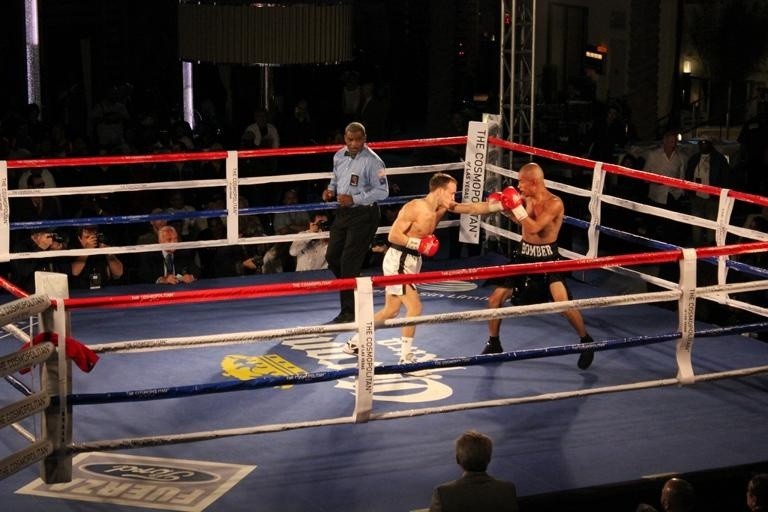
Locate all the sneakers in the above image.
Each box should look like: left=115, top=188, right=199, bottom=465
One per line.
left=481, top=341, right=502, bottom=355
left=343, top=339, right=358, bottom=355
left=577, top=350, right=594, bottom=370
left=333, top=312, right=354, bottom=322
left=398, top=358, right=425, bottom=377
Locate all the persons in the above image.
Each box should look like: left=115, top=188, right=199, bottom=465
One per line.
left=428, top=428, right=519, bottom=511
left=744, top=471, right=768, bottom=511
left=0, top=68, right=767, bottom=290
left=476, top=160, right=599, bottom=370
left=660, top=473, right=695, bottom=511
left=321, top=122, right=390, bottom=327
left=343, top=171, right=509, bottom=371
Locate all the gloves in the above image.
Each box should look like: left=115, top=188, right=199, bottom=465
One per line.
left=405, top=235, right=439, bottom=256
left=487, top=186, right=528, bottom=222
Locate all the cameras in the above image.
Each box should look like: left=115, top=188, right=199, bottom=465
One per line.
left=51, top=233, right=65, bottom=242
left=94, top=231, right=104, bottom=244
left=313, top=220, right=330, bottom=230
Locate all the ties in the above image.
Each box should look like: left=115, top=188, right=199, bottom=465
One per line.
left=167, top=253, right=173, bottom=274
